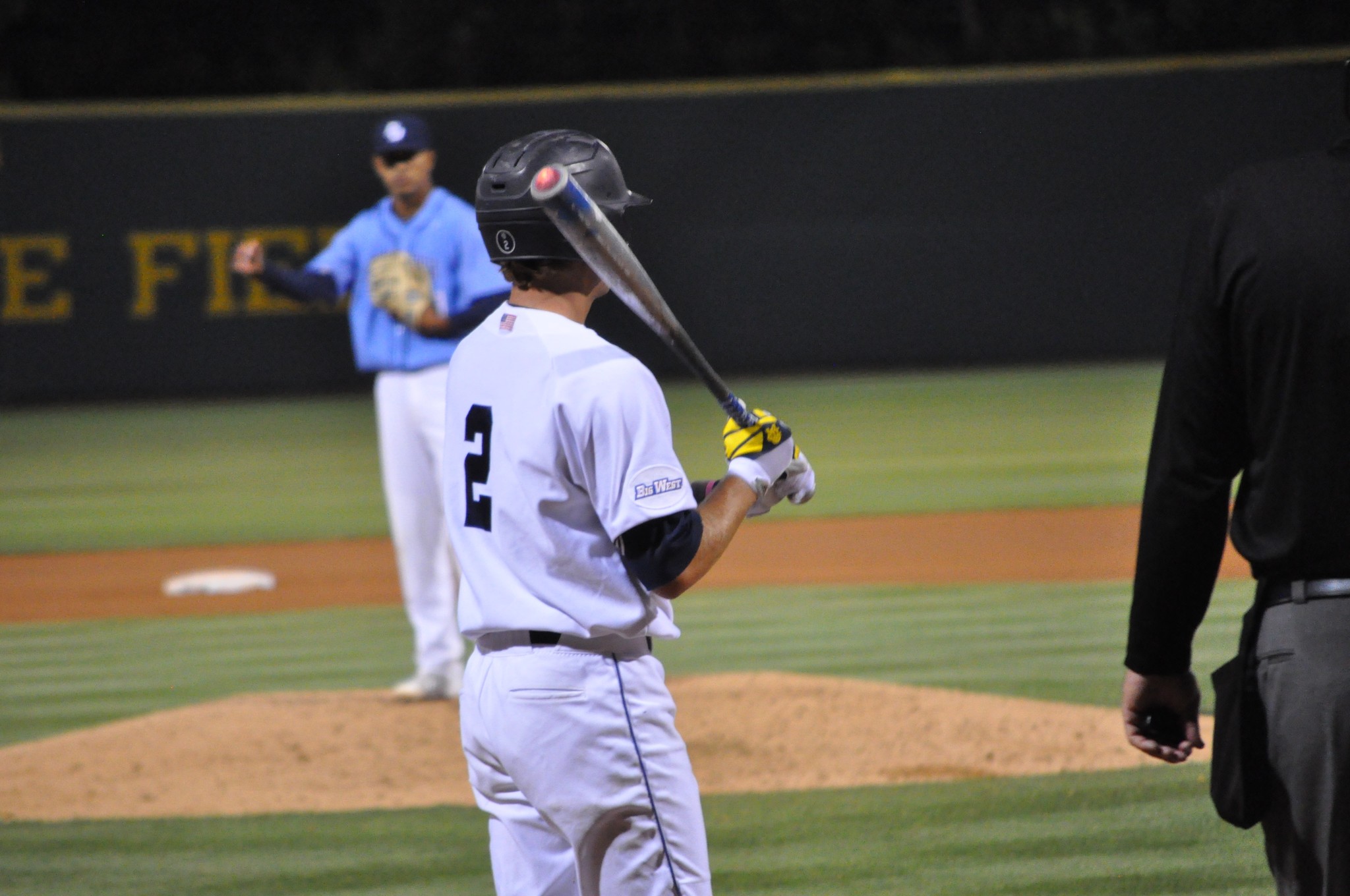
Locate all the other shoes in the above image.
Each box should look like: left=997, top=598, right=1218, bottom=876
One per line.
left=394, top=678, right=443, bottom=698
left=443, top=671, right=461, bottom=697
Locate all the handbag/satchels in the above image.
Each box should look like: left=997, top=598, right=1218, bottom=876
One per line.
left=1211, top=605, right=1273, bottom=830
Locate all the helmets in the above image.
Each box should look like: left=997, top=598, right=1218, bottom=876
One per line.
left=476, top=129, right=654, bottom=263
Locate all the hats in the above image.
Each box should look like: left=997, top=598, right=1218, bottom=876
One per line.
left=371, top=115, right=432, bottom=155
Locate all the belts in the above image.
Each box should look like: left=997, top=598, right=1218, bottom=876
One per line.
left=527, top=630, right=654, bottom=651
left=1253, top=576, right=1349, bottom=606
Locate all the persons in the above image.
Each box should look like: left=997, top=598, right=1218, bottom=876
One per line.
left=1121, top=147, right=1350, bottom=896
left=230, top=112, right=514, bottom=701
left=439, top=127, right=817, bottom=896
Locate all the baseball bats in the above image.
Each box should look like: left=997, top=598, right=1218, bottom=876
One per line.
left=531, top=162, right=758, bottom=431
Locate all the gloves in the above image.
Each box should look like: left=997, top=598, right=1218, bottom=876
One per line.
left=744, top=446, right=814, bottom=517
left=723, top=397, right=796, bottom=495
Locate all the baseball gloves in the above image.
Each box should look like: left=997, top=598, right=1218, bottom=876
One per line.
left=370, top=250, right=437, bottom=333
